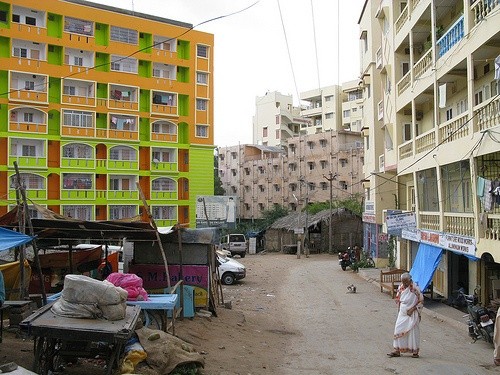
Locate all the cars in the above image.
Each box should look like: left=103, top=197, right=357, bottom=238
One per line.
left=216, top=252, right=246, bottom=285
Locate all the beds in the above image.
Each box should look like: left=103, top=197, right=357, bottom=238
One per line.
left=379, top=270, right=434, bottom=300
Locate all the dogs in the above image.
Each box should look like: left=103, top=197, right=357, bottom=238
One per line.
left=348, top=283, right=356, bottom=293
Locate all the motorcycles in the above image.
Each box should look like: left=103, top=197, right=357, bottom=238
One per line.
left=460, top=289, right=495, bottom=345
left=338, top=249, right=355, bottom=271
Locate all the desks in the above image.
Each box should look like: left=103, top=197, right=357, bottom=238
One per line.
left=3, top=301, right=32, bottom=328
left=18, top=302, right=141, bottom=375
left=46, top=291, right=178, bottom=337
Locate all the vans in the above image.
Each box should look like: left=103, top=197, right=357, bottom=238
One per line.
left=218, top=233, right=247, bottom=258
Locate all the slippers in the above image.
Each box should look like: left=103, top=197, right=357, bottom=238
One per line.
left=412, top=354, right=419, bottom=358
left=386, top=352, right=401, bottom=357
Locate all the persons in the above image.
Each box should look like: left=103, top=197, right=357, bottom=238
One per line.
left=387, top=272, right=424, bottom=358
left=493, top=307, right=500, bottom=366
